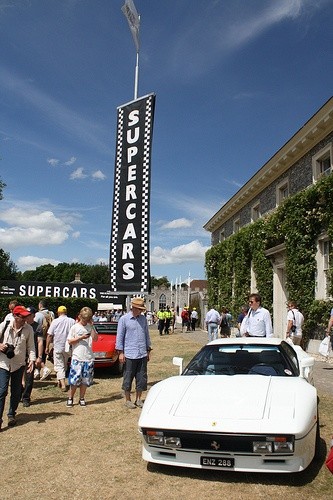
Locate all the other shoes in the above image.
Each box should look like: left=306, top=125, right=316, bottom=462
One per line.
left=125, top=399, right=136, bottom=409
left=134, top=399, right=144, bottom=408
left=66, top=397, right=74, bottom=408
left=57, top=383, right=67, bottom=392
left=7, top=414, right=17, bottom=427
left=23, top=398, right=31, bottom=407
left=78, top=398, right=86, bottom=407
left=40, top=367, right=52, bottom=382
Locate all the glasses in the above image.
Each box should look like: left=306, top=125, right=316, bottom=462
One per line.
left=249, top=300, right=255, bottom=304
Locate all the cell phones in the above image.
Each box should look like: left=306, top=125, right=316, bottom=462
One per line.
left=86, top=333, right=90, bottom=335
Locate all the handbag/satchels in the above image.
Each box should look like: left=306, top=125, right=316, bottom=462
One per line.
left=318, top=336, right=330, bottom=357
left=290, top=324, right=296, bottom=332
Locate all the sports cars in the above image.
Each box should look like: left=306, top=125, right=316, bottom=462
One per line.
left=140, top=337, right=319, bottom=474
left=67, top=322, right=125, bottom=374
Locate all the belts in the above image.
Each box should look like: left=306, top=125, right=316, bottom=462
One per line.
left=210, top=322, right=217, bottom=323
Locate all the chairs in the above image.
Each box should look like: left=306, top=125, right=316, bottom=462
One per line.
left=259, top=350, right=287, bottom=375
left=205, top=352, right=235, bottom=375
left=231, top=350, right=254, bottom=374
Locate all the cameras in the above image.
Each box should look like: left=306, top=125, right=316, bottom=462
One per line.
left=291, top=325, right=296, bottom=332
left=2, top=343, right=15, bottom=359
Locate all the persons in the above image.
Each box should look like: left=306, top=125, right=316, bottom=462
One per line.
left=285, top=300, right=304, bottom=345
left=326, top=308, right=333, bottom=352
left=115, top=297, right=151, bottom=408
left=67, top=306, right=99, bottom=408
left=206, top=304, right=247, bottom=342
left=0, top=300, right=77, bottom=431
left=240, top=294, right=272, bottom=338
left=147, top=307, right=198, bottom=336
left=94, top=308, right=128, bottom=323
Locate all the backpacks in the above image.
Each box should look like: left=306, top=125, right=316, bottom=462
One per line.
left=39, top=310, right=54, bottom=336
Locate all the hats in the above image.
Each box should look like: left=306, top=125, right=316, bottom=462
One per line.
left=56, top=306, right=67, bottom=313
left=129, top=298, right=147, bottom=310
left=193, top=308, right=196, bottom=311
left=13, top=306, right=31, bottom=317
